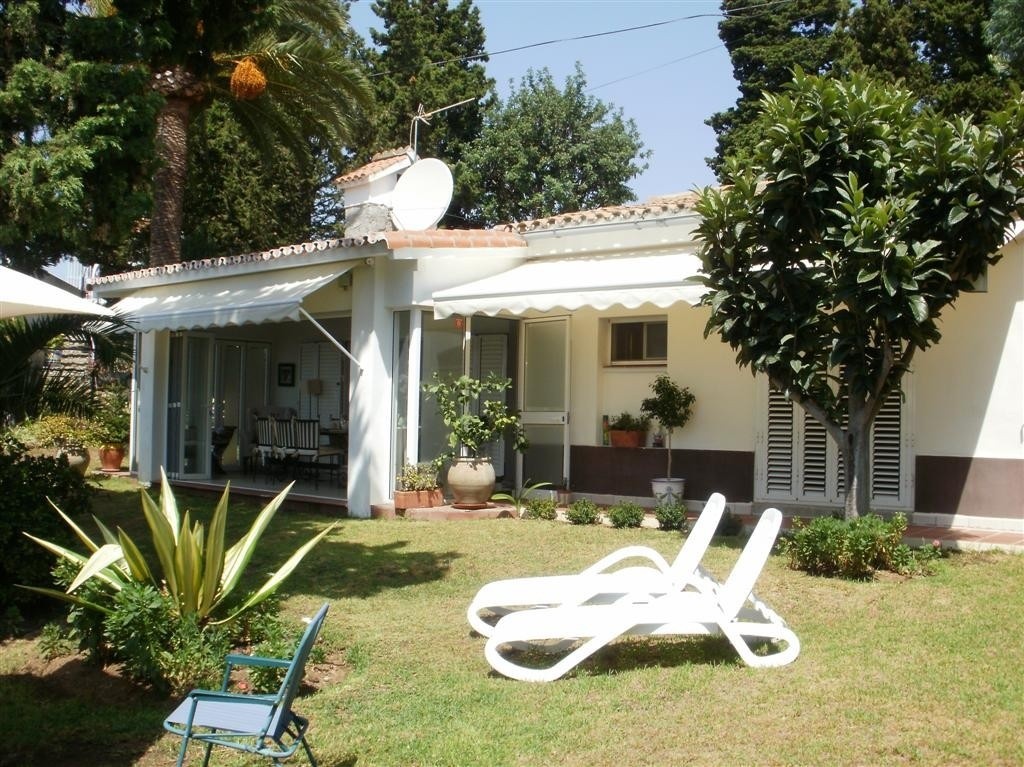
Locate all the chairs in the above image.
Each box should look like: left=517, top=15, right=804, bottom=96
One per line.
left=465, top=491, right=804, bottom=688
left=254, top=409, right=342, bottom=490
left=162, top=599, right=331, bottom=767
left=181, top=419, right=240, bottom=481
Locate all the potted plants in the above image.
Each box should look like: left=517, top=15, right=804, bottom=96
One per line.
left=420, top=366, right=532, bottom=510
left=639, top=372, right=699, bottom=511
left=93, top=391, right=131, bottom=472
left=394, top=455, right=446, bottom=512
left=606, top=411, right=651, bottom=447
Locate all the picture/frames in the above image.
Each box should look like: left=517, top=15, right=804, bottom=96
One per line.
left=277, top=364, right=298, bottom=388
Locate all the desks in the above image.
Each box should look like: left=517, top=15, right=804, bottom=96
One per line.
left=317, top=426, right=349, bottom=485
